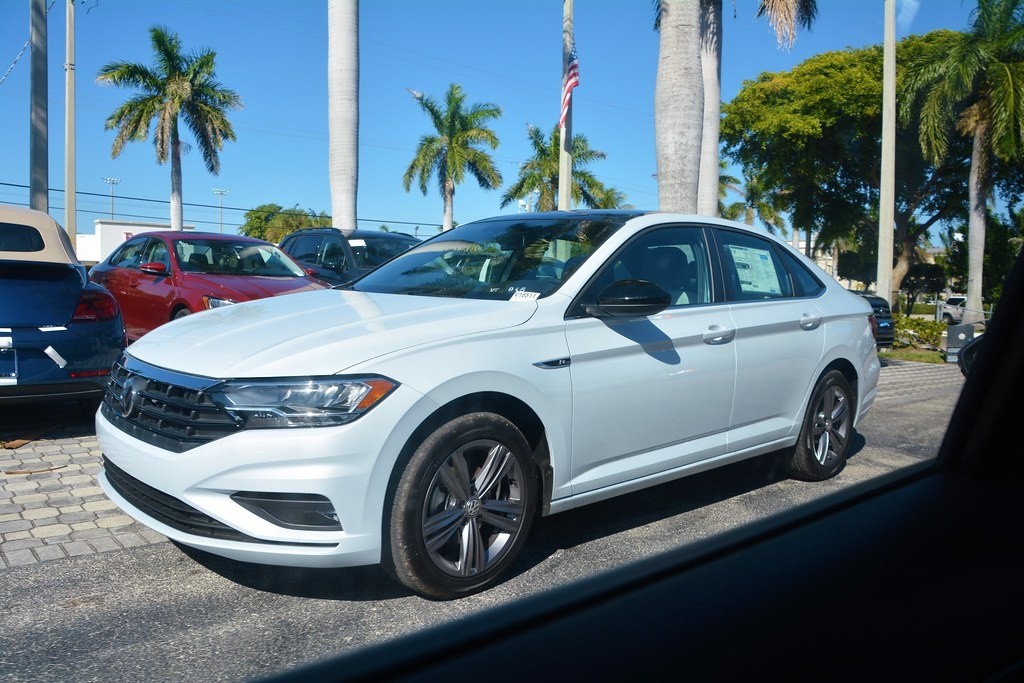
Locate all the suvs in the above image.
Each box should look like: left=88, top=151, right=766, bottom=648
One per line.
left=277, top=227, right=460, bottom=291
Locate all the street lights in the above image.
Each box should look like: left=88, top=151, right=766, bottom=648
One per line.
left=210, top=188, right=230, bottom=234
left=102, top=177, right=122, bottom=220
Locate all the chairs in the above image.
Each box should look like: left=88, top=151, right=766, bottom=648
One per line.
left=134, top=251, right=153, bottom=264
left=189, top=253, right=210, bottom=273
left=513, top=248, right=695, bottom=309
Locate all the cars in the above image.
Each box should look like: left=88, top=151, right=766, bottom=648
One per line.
left=846, top=289, right=896, bottom=349
left=85, top=230, right=332, bottom=345
left=927, top=296, right=968, bottom=325
left=92, top=210, right=883, bottom=602
left=0, top=202, right=129, bottom=405
